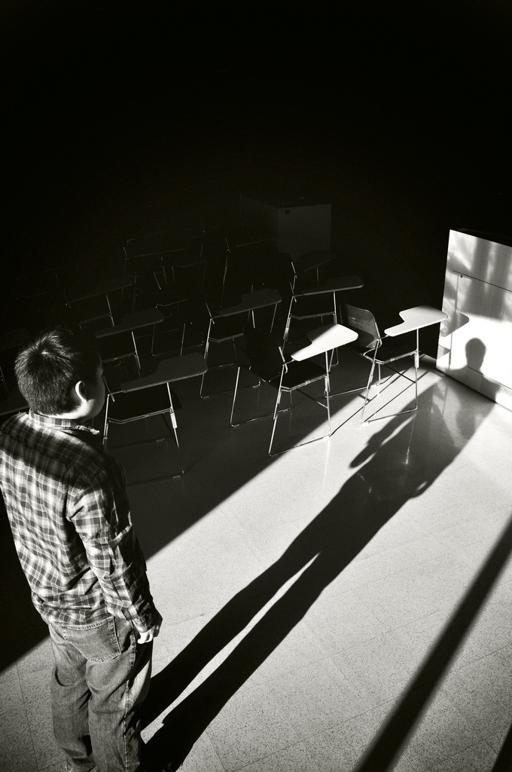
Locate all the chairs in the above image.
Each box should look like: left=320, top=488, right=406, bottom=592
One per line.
left=1, top=258, right=448, bottom=486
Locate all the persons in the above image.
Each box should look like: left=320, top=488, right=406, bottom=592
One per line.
left=1, top=322, right=166, bottom=772
